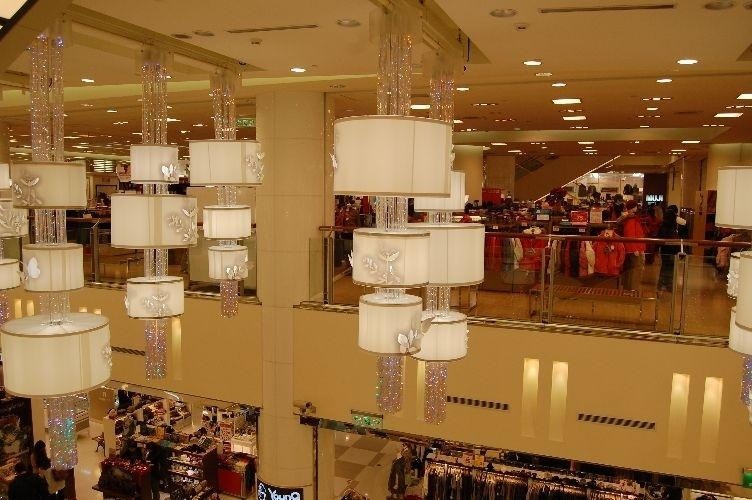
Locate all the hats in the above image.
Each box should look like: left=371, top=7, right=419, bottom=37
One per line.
left=626, top=200, right=637, bottom=210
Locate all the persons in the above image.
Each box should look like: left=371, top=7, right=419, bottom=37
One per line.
left=36, top=456, right=70, bottom=499
left=31, top=438, right=47, bottom=474
left=146, top=439, right=163, bottom=497
left=618, top=201, right=644, bottom=291
left=6, top=461, right=50, bottom=500
left=116, top=389, right=130, bottom=412
left=116, top=436, right=144, bottom=460
left=639, top=202, right=690, bottom=292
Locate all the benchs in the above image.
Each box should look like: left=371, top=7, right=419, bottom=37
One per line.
left=529, top=284, right=660, bottom=332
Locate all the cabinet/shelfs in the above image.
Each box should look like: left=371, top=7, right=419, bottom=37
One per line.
left=0, top=399, right=36, bottom=477
left=91, top=389, right=258, bottom=500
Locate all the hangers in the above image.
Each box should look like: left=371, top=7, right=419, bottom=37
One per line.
left=523, top=216, right=540, bottom=234
left=421, top=456, right=645, bottom=500
left=602, top=225, right=616, bottom=237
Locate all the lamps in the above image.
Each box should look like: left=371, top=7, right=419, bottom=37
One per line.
left=1, top=29, right=114, bottom=469
left=111, top=45, right=198, bottom=379
left=714, top=166, right=751, bottom=425
left=331, top=1, right=454, bottom=411
left=186, top=68, right=265, bottom=318
left=405, top=44, right=486, bottom=427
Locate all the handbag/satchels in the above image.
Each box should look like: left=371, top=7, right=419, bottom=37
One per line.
left=51, top=469, right=68, bottom=481
